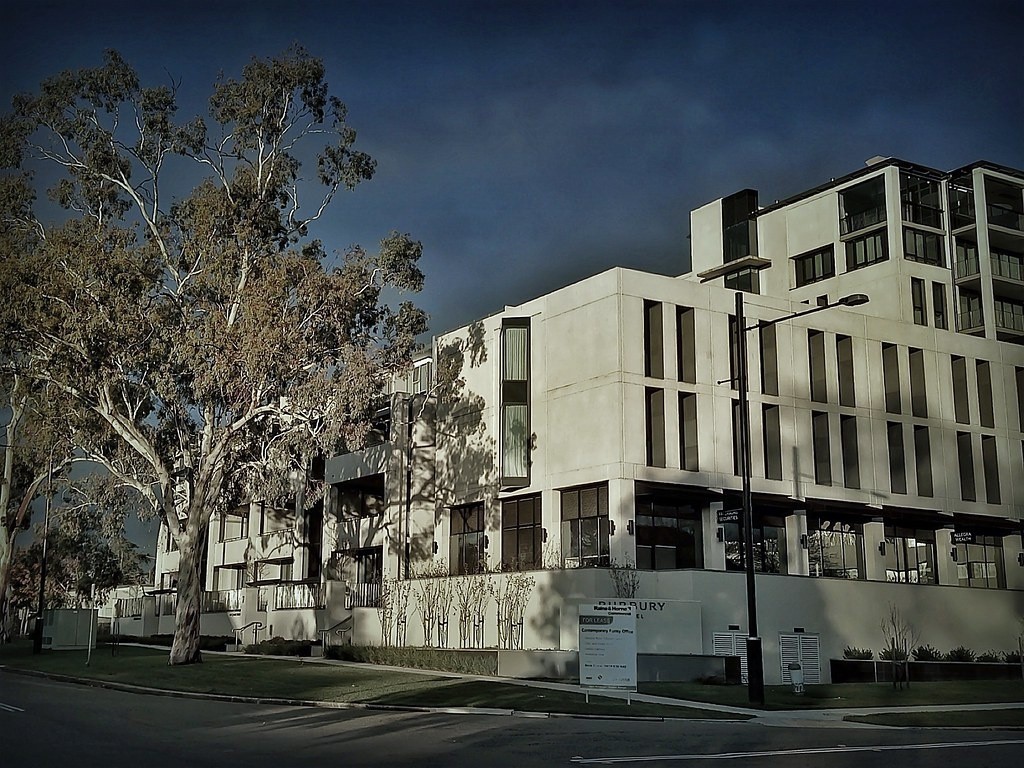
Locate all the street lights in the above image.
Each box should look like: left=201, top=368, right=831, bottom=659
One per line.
left=716, top=292, right=871, bottom=708
